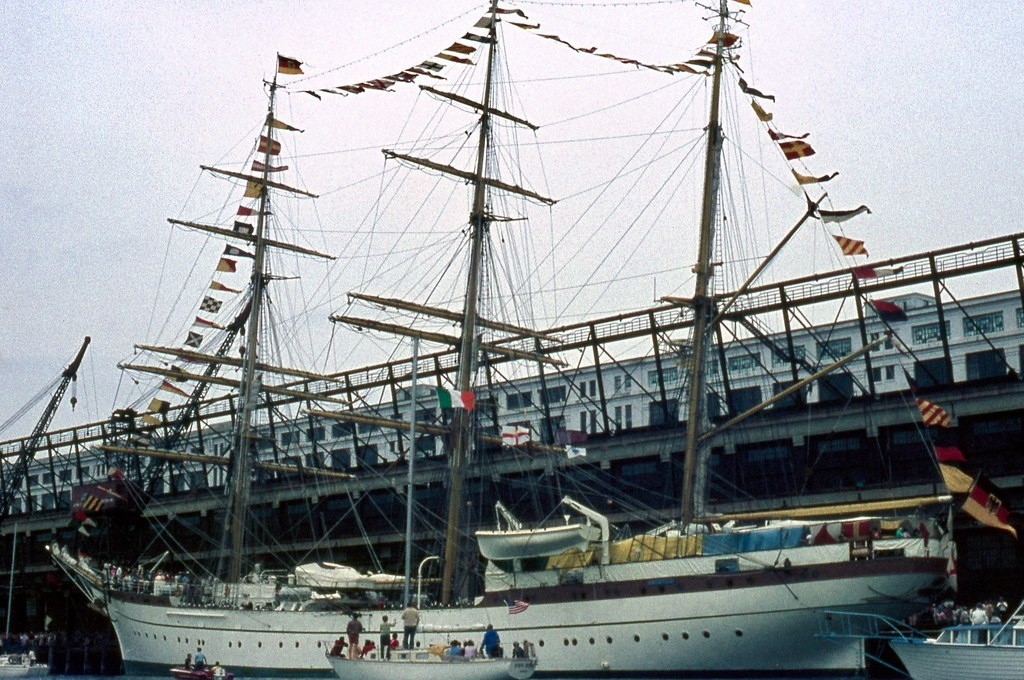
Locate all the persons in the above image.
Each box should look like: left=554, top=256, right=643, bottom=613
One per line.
left=800, top=527, right=912, bottom=545
left=928, top=596, right=1008, bottom=626
left=102, top=563, right=217, bottom=605
left=185, top=647, right=223, bottom=673
left=2, top=630, right=106, bottom=647
left=328, top=602, right=524, bottom=659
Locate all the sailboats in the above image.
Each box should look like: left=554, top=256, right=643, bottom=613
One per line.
left=49, top=0, right=958, bottom=680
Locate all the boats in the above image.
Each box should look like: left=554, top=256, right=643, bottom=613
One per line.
left=812, top=608, right=1024, bottom=680
left=474, top=525, right=601, bottom=560
left=0, top=650, right=49, bottom=677
left=169, top=666, right=234, bottom=680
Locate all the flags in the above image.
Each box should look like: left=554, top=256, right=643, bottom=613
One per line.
left=144, top=258, right=237, bottom=414
left=393, top=71, right=419, bottom=81
left=871, top=299, right=908, bottom=322
left=445, top=42, right=476, bottom=55
left=337, top=85, right=365, bottom=95
left=539, top=27, right=742, bottom=77
left=504, top=599, right=529, bottom=615
left=233, top=220, right=254, bottom=235
left=916, top=396, right=1018, bottom=535
left=739, top=79, right=816, bottom=161
left=83, top=466, right=123, bottom=511
left=437, top=385, right=586, bottom=459
left=240, top=54, right=305, bottom=198
left=416, top=60, right=446, bottom=71
left=140, top=414, right=161, bottom=426
left=474, top=16, right=502, bottom=28
left=367, top=78, right=395, bottom=89
left=835, top=237, right=871, bottom=258
